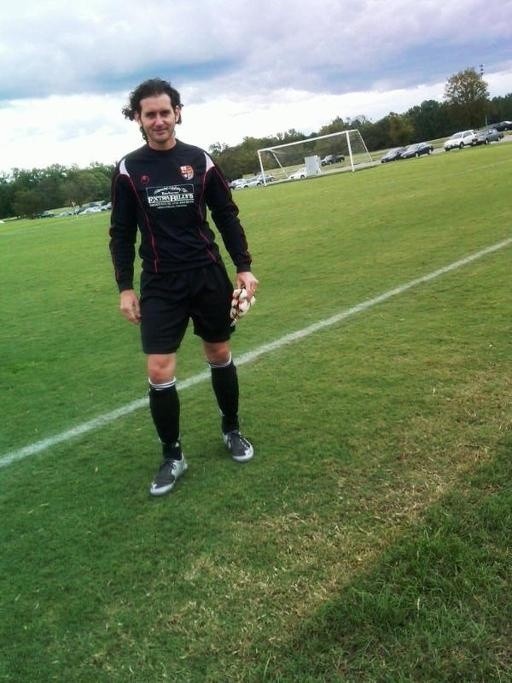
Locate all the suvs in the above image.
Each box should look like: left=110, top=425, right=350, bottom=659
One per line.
left=444, top=129, right=478, bottom=151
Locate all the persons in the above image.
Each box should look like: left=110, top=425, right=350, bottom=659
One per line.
left=107, top=74, right=262, bottom=498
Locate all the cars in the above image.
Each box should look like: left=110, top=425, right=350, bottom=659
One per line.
left=471, top=128, right=504, bottom=146
left=229, top=166, right=321, bottom=192
left=321, top=154, right=344, bottom=166
left=494, top=120, right=512, bottom=131
left=381, top=147, right=407, bottom=163
left=401, top=142, right=434, bottom=159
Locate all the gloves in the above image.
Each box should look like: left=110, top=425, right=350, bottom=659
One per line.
left=230, top=288, right=255, bottom=327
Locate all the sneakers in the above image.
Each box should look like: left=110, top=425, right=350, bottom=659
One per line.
left=222, top=429, right=254, bottom=462
left=149, top=450, right=188, bottom=496
